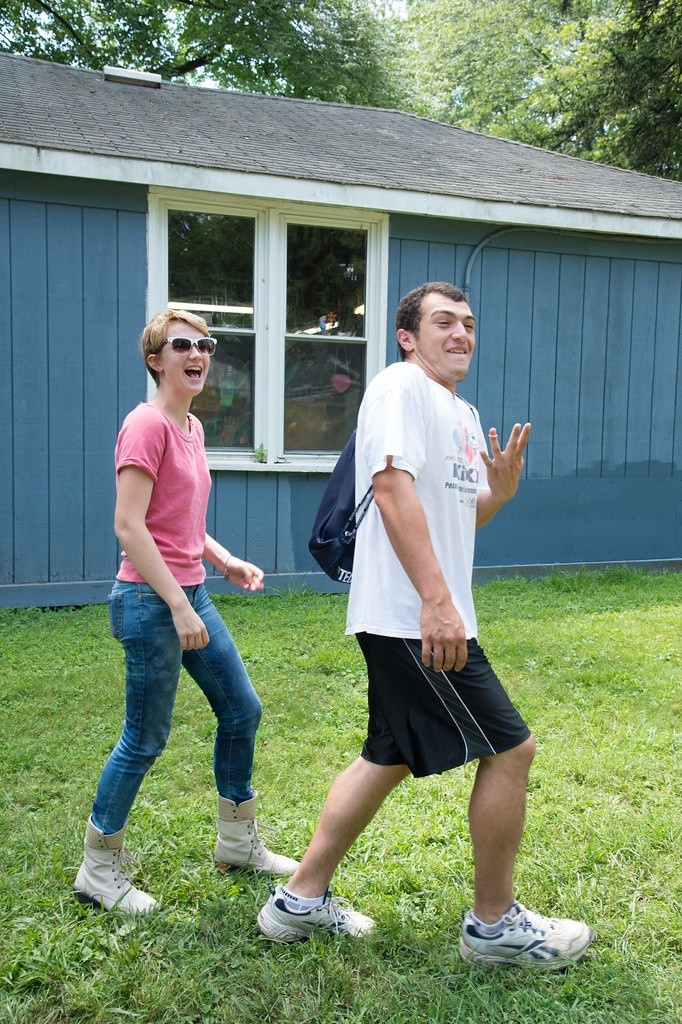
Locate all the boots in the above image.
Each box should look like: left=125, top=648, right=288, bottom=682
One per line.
left=72, top=812, right=161, bottom=915
left=215, top=791, right=300, bottom=876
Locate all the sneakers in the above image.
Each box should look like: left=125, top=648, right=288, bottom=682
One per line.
left=459, top=901, right=590, bottom=972
left=258, top=882, right=377, bottom=943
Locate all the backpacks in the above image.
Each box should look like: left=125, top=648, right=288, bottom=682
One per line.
left=308, top=427, right=376, bottom=583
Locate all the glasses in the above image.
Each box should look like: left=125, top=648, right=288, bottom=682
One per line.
left=155, top=337, right=219, bottom=357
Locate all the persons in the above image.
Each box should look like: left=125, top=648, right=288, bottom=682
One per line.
left=72, top=309, right=300, bottom=913
left=257, top=281, right=593, bottom=970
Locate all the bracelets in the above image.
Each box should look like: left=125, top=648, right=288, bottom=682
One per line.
left=223, top=555, right=232, bottom=581
